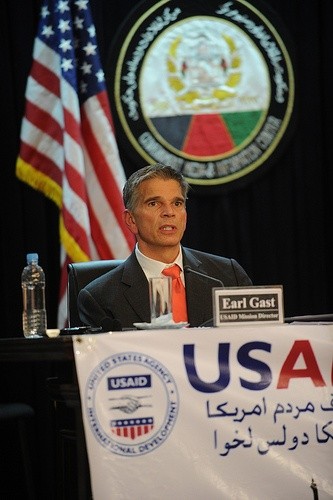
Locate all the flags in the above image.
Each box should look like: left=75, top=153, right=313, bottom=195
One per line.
left=16, top=0, right=137, bottom=329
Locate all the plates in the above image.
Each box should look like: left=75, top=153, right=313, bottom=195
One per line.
left=133, top=322, right=190, bottom=330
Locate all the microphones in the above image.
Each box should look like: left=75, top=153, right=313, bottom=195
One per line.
left=183, top=265, right=225, bottom=287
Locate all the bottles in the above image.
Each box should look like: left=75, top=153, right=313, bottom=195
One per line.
left=21, top=253, right=47, bottom=339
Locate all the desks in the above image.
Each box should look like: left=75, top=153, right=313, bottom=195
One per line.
left=0, top=324, right=333, bottom=500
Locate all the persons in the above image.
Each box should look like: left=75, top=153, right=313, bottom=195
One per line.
left=76, top=163, right=254, bottom=332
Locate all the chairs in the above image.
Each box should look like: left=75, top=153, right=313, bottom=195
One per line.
left=45, top=260, right=119, bottom=500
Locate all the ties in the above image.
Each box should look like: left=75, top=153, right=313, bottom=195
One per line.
left=162, top=265, right=188, bottom=325
